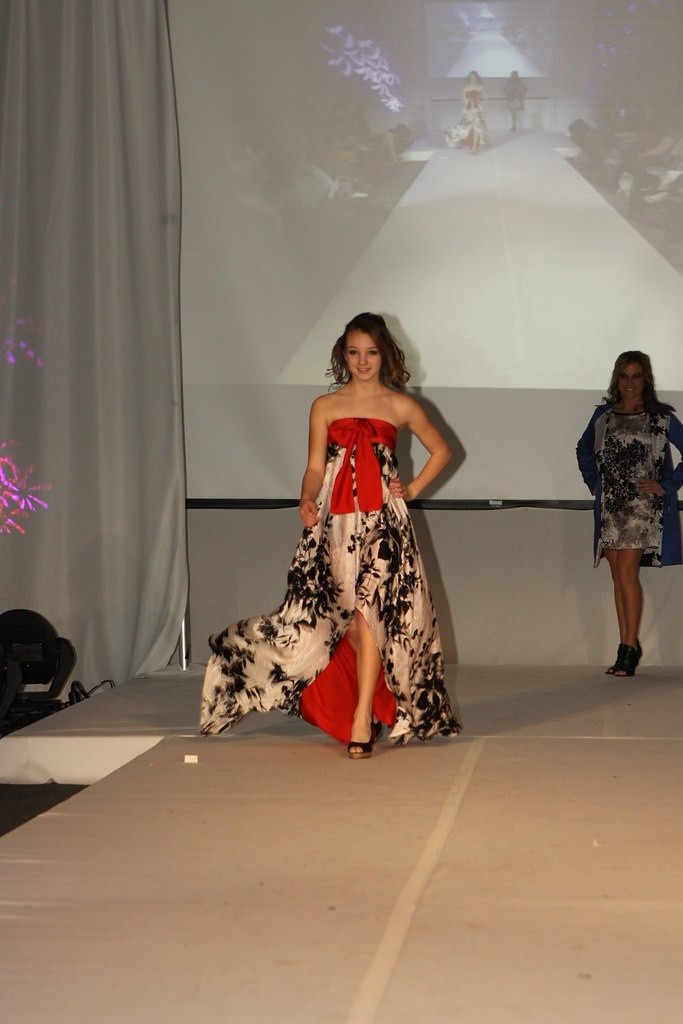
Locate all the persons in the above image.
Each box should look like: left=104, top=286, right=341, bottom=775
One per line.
left=444, top=72, right=489, bottom=155
left=201, top=117, right=413, bottom=352
left=575, top=351, right=683, bottom=677
left=568, top=104, right=683, bottom=267
left=503, top=70, right=526, bottom=135
left=197, top=312, right=462, bottom=759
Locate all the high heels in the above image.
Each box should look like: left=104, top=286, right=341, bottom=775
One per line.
left=605, top=643, right=628, bottom=674
left=347, top=714, right=383, bottom=759
left=614, top=639, right=642, bottom=676
